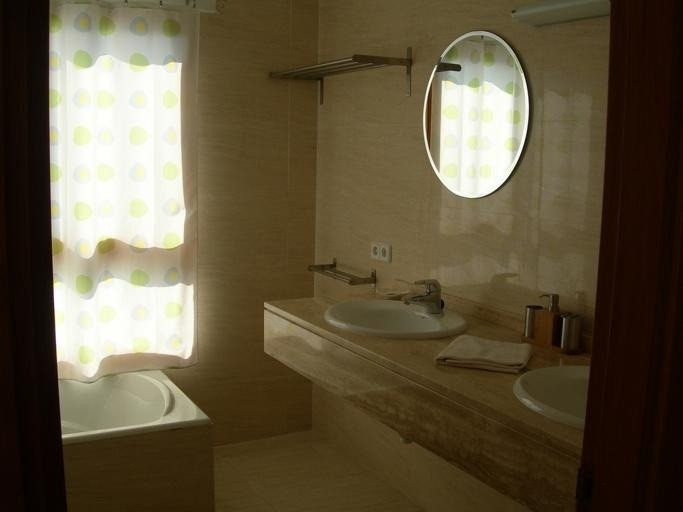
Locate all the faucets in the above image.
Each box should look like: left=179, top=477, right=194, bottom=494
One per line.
left=404, top=280, right=446, bottom=314
left=489, top=273, right=521, bottom=294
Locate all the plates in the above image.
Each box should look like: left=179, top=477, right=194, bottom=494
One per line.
left=375, top=286, right=411, bottom=298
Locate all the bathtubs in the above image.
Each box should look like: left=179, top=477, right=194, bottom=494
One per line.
left=57, top=370, right=229, bottom=447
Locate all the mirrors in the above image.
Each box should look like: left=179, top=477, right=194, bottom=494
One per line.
left=422, top=30, right=530, bottom=198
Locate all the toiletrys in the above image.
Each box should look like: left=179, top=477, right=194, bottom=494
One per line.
left=571, top=290, right=590, bottom=319
left=534, top=294, right=566, bottom=349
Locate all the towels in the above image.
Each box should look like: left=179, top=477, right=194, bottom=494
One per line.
left=434, top=334, right=536, bottom=373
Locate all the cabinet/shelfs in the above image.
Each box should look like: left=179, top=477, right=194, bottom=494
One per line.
left=269, top=47, right=412, bottom=298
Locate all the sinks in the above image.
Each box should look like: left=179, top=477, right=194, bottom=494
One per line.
left=325, top=298, right=468, bottom=340
left=513, top=364, right=590, bottom=430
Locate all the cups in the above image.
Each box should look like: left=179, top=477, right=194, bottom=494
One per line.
left=561, top=315, right=582, bottom=356
left=524, top=305, right=543, bottom=339
left=534, top=309, right=553, bottom=348
